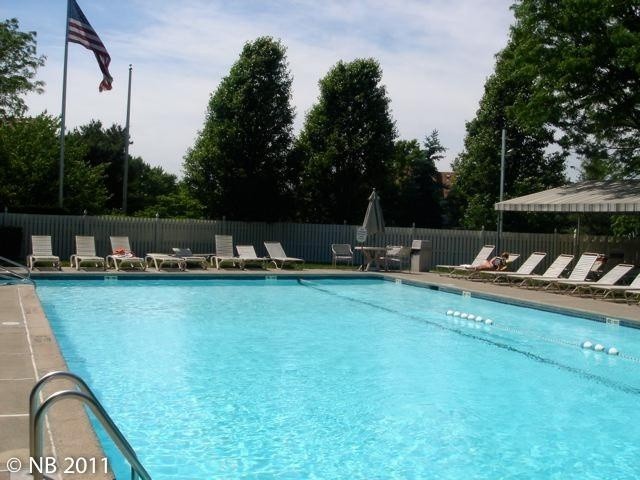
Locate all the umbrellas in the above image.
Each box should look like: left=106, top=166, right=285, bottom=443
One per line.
left=363, top=187, right=388, bottom=258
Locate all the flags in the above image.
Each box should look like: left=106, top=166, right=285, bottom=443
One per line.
left=66, top=0, right=115, bottom=92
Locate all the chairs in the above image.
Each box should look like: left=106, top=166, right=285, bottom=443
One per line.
left=208, top=232, right=241, bottom=272
left=171, top=246, right=207, bottom=271
left=264, top=241, right=306, bottom=271
left=324, top=234, right=640, bottom=309
left=102, top=234, right=146, bottom=273
left=26, top=234, right=62, bottom=272
left=144, top=252, right=184, bottom=273
left=69, top=235, right=107, bottom=273
left=236, top=244, right=269, bottom=271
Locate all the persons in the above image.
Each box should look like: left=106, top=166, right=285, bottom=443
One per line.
left=465, top=251, right=511, bottom=272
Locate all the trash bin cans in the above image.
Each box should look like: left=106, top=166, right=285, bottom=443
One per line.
left=410, top=240, right=433, bottom=272
left=0, top=227, right=23, bottom=267
